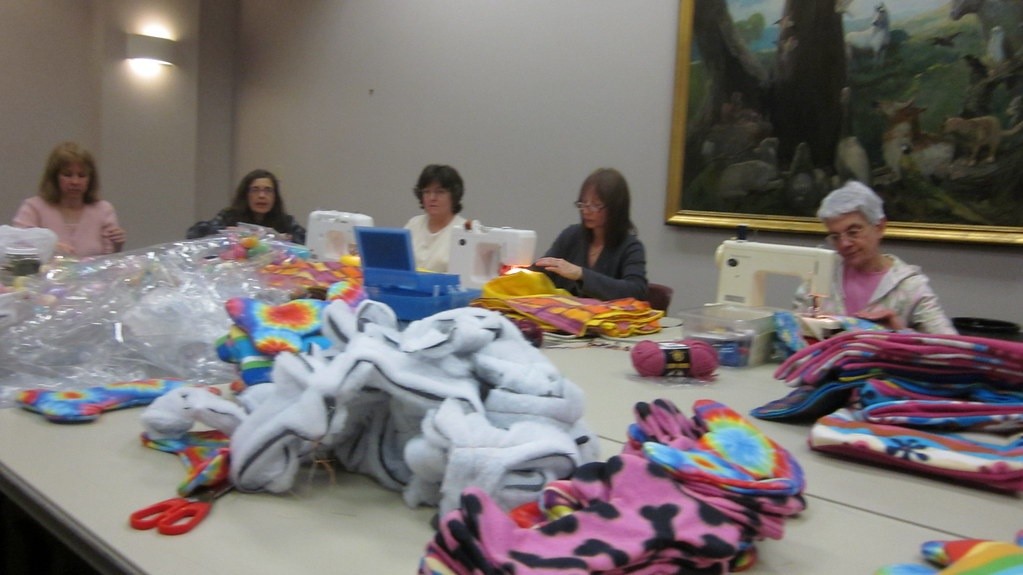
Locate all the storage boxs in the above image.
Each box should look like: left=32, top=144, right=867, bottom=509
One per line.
left=678, top=303, right=773, bottom=369
left=354, top=225, right=486, bottom=322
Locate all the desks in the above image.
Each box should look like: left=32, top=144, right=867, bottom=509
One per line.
left=0, top=318, right=1023, bottom=575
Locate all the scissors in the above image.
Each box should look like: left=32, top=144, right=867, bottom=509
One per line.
left=127, top=479, right=236, bottom=537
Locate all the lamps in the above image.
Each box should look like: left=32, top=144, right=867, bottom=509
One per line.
left=125, top=22, right=184, bottom=82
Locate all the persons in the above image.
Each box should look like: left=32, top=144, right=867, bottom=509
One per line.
left=11, top=140, right=128, bottom=256
left=185, top=169, right=306, bottom=245
left=401, top=164, right=473, bottom=272
left=789, top=180, right=959, bottom=335
left=465, top=167, right=649, bottom=301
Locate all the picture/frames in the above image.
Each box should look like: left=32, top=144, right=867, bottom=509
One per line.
left=664, top=0, right=1023, bottom=247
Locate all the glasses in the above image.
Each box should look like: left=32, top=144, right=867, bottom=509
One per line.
left=574, top=200, right=606, bottom=212
left=825, top=224, right=872, bottom=245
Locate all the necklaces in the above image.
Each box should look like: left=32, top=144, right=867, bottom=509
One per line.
left=589, top=250, right=597, bottom=255
